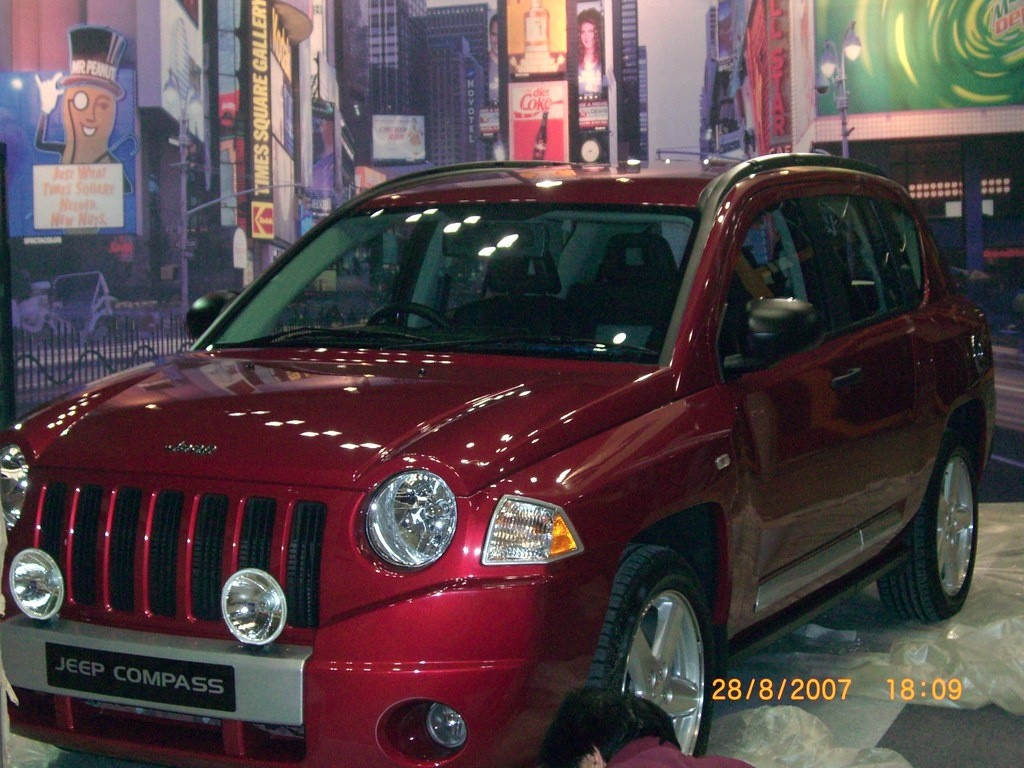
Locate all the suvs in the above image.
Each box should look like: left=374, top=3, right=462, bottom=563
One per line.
left=0, top=153, right=999, bottom=768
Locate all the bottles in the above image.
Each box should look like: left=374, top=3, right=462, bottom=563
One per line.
left=523, top=1, right=549, bottom=60
left=533, top=111, right=548, bottom=162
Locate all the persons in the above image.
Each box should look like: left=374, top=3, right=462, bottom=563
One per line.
left=537, top=685, right=755, bottom=768
left=574, top=9, right=603, bottom=96
left=487, top=15, right=499, bottom=101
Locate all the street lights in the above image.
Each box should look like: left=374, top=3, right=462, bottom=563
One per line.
left=163, top=68, right=204, bottom=328
left=813, top=20, right=862, bottom=158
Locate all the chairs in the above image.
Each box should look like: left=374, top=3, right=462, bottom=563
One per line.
left=576, top=230, right=691, bottom=350
left=451, top=224, right=590, bottom=340
